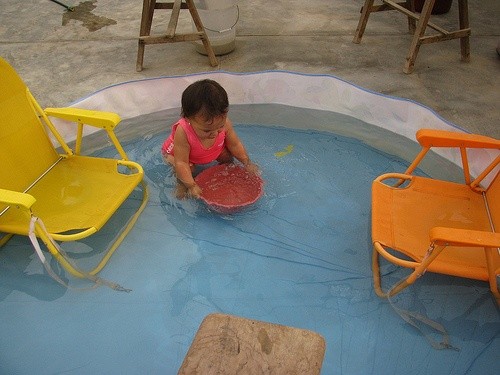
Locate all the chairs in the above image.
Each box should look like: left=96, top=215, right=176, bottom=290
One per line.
left=173, top=314, right=326, bottom=374
left=0, top=59, right=149, bottom=278
left=366, top=129, right=499, bottom=313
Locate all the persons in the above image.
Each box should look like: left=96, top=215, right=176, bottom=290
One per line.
left=161, top=79, right=262, bottom=199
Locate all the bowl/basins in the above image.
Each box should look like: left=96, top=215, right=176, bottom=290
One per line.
left=193, top=163, right=264, bottom=214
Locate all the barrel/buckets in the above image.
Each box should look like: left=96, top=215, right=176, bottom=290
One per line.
left=190, top=0, right=237, bottom=56
